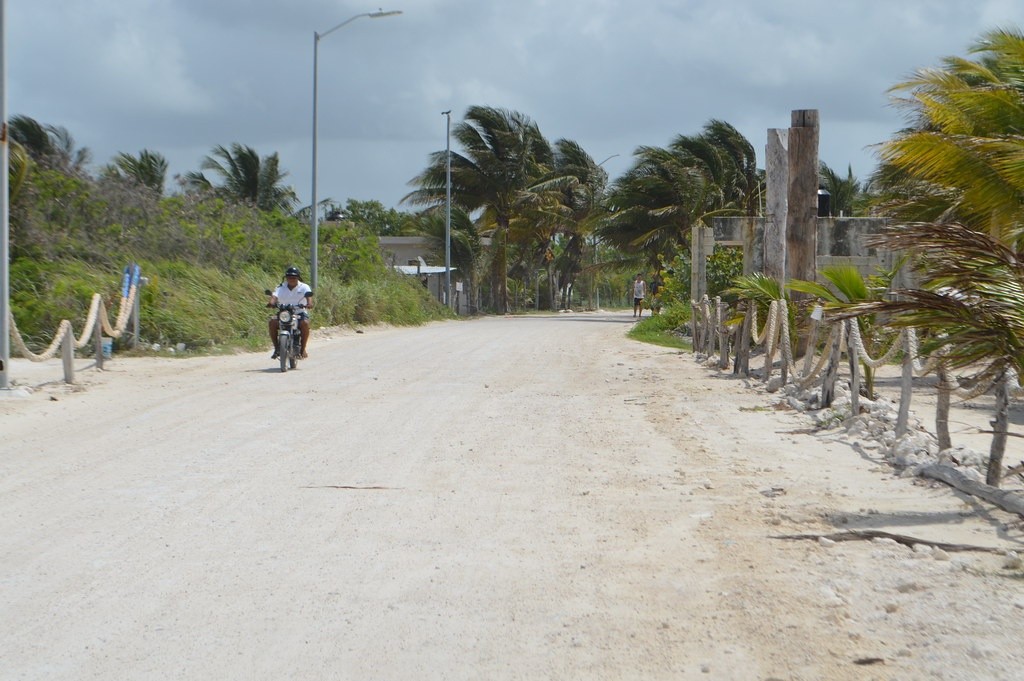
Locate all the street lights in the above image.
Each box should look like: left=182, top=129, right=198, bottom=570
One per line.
left=440, top=109, right=453, bottom=309
left=310, top=7, right=402, bottom=295
left=593, top=152, right=616, bottom=265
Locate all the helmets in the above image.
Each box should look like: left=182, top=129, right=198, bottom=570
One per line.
left=285, top=265, right=300, bottom=276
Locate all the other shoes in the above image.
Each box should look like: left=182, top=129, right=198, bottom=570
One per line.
left=271, top=349, right=280, bottom=359
left=300, top=349, right=308, bottom=358
left=633, top=315, right=636, bottom=318
left=639, top=314, right=641, bottom=317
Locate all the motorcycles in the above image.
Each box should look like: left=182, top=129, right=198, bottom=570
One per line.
left=264, top=289, right=314, bottom=372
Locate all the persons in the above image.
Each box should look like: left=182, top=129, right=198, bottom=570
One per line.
left=633, top=274, right=662, bottom=317
left=268, top=266, right=314, bottom=360
